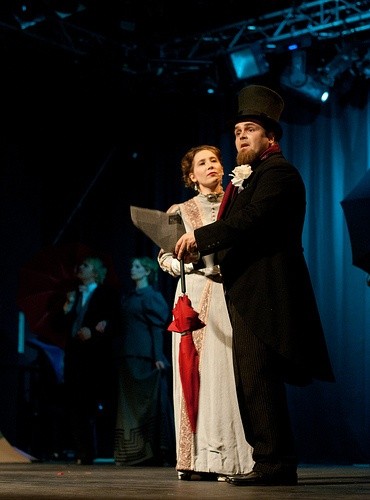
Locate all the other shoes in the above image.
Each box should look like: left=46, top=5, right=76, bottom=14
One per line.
left=177, top=470, right=203, bottom=481
left=218, top=475, right=227, bottom=481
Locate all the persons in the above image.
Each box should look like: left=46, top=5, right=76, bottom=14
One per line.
left=175, top=83, right=337, bottom=486
left=157, top=146, right=255, bottom=481
left=46, top=255, right=122, bottom=464
left=114, top=257, right=173, bottom=468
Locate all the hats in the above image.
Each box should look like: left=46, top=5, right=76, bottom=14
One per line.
left=231, top=85, right=285, bottom=142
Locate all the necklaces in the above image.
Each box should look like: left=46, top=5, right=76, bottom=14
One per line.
left=197, top=190, right=222, bottom=202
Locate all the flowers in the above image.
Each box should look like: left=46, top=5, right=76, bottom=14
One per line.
left=228, top=164, right=253, bottom=193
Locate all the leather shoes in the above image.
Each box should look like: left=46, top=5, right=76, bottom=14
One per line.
left=225, top=471, right=298, bottom=486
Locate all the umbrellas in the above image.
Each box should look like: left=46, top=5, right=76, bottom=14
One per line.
left=167, top=249, right=207, bottom=456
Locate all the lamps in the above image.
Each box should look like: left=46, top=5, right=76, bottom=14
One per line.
left=154, top=43, right=370, bottom=108
